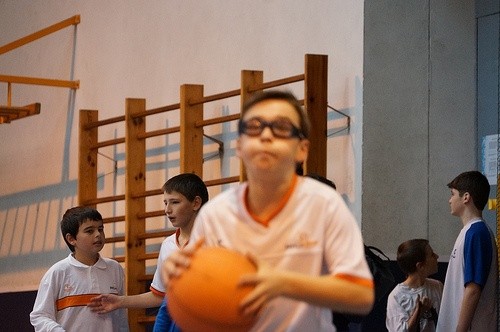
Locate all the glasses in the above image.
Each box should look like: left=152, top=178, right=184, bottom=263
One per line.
left=241, top=118, right=306, bottom=140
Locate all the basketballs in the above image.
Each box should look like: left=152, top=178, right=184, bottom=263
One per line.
left=167, top=246, right=261, bottom=332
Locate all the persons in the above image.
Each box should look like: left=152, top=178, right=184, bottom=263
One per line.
left=86, top=172, right=209, bottom=332
left=159, top=91, right=375, bottom=332
left=303, top=170, right=500, bottom=332
left=29, top=207, right=130, bottom=332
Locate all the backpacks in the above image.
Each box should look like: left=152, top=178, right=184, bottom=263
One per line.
left=364, top=244, right=400, bottom=298
